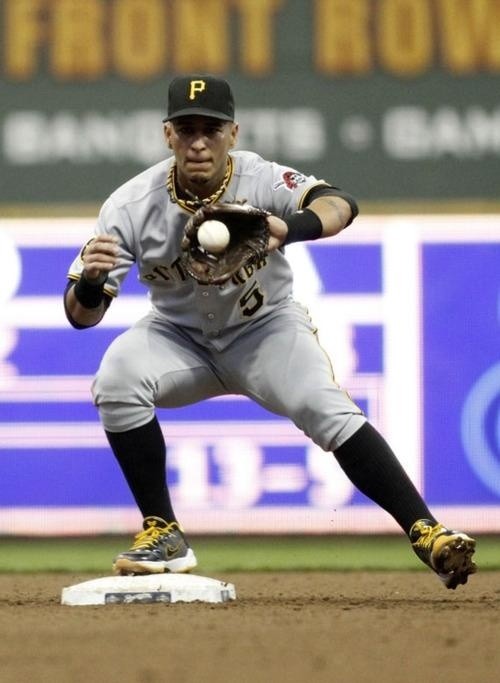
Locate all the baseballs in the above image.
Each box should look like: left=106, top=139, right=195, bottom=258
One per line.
left=197, top=220, right=230, bottom=252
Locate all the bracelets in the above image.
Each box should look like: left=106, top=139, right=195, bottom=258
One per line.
left=280, top=207, right=323, bottom=247
left=73, top=268, right=109, bottom=311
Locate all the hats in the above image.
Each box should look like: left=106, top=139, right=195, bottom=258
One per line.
left=162, top=75, right=234, bottom=123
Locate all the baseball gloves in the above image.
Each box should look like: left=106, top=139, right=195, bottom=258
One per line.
left=181, top=202, right=273, bottom=284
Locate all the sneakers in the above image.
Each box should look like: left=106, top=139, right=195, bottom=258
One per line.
left=410, top=519, right=477, bottom=589
left=114, top=516, right=197, bottom=576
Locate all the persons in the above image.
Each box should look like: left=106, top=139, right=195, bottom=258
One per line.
left=62, top=72, right=479, bottom=591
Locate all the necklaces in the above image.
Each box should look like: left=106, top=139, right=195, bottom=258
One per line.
left=164, top=151, right=232, bottom=207
